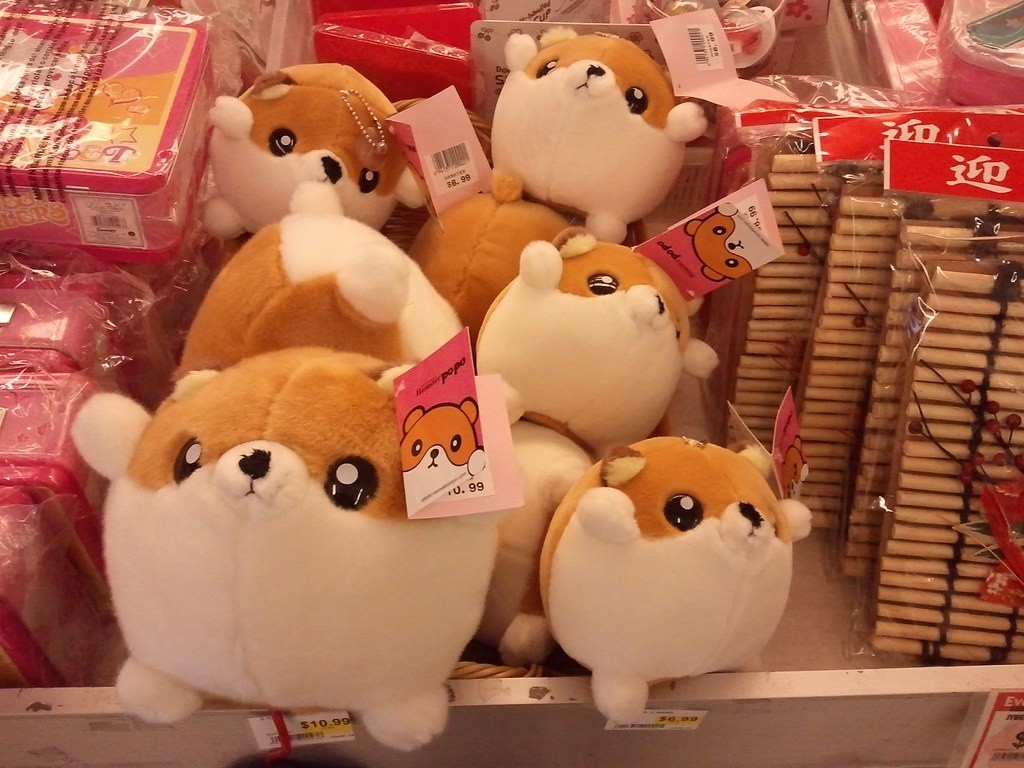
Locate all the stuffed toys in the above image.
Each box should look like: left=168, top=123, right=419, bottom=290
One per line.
left=539, top=436, right=814, bottom=725
left=202, top=63, right=428, bottom=241
left=476, top=226, right=721, bottom=460
left=69, top=343, right=503, bottom=754
left=408, top=173, right=573, bottom=342
left=475, top=421, right=600, bottom=668
left=181, top=180, right=463, bottom=375
left=490, top=26, right=707, bottom=244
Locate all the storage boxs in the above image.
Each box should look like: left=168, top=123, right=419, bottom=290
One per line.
left=0, top=0, right=217, bottom=263
left=0, top=486, right=100, bottom=689
left=0, top=270, right=118, bottom=374
left=0, top=368, right=116, bottom=624
left=861, top=0, right=961, bottom=106
left=935, top=0, right=1024, bottom=107
